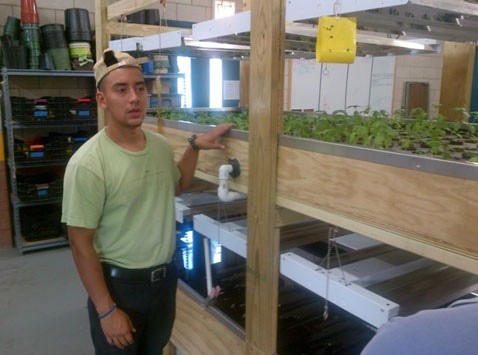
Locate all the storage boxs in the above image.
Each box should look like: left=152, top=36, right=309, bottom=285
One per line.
left=147, top=94, right=184, bottom=108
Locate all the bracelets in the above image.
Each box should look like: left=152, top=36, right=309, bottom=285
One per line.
left=188, top=135, right=200, bottom=152
left=99, top=303, right=116, bottom=320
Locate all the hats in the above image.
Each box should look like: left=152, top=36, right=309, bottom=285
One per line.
left=93, top=48, right=142, bottom=88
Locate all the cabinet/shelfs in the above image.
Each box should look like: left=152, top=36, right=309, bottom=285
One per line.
left=0, top=69, right=105, bottom=256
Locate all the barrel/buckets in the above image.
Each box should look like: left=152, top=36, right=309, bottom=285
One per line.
left=39, top=23, right=71, bottom=71
left=145, top=7, right=160, bottom=25
left=125, top=9, right=145, bottom=25
left=63, top=8, right=92, bottom=42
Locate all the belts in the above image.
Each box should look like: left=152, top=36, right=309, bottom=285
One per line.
left=101, top=262, right=175, bottom=283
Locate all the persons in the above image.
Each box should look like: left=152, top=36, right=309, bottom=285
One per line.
left=360, top=297, right=477, bottom=355
left=61, top=49, right=240, bottom=355
